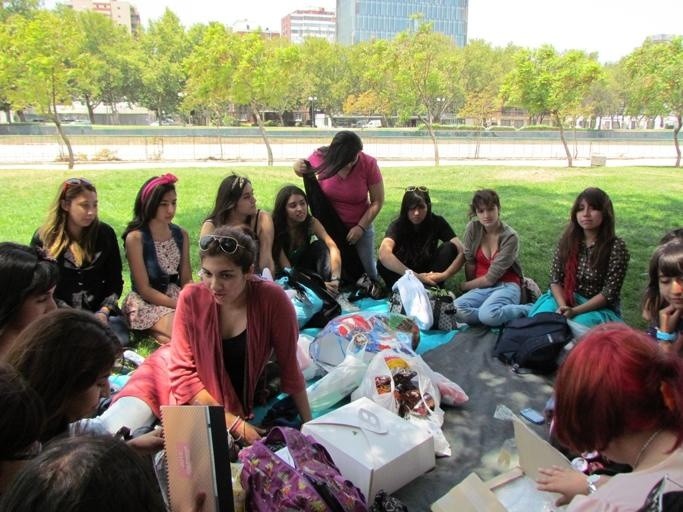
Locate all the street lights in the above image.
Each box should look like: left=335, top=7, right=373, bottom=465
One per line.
left=308, top=96, right=317, bottom=127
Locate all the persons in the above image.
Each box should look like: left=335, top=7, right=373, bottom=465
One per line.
left=528, top=188, right=631, bottom=339
left=642, top=229, right=683, bottom=343
left=535, top=323, right=683, bottom=512
left=272, top=186, right=344, bottom=299
left=30, top=177, right=130, bottom=347
left=121, top=174, right=193, bottom=346
left=377, top=187, right=466, bottom=299
left=68, top=224, right=312, bottom=464
left=451, top=191, right=534, bottom=327
left=1, top=308, right=125, bottom=501
left=0, top=241, right=61, bottom=357
left=198, top=171, right=275, bottom=281
left=0, top=427, right=171, bottom=512
left=293, top=132, right=385, bottom=297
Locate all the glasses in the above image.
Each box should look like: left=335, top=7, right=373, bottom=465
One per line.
left=64, top=178, right=90, bottom=195
left=406, top=186, right=429, bottom=193
left=199, top=235, right=246, bottom=255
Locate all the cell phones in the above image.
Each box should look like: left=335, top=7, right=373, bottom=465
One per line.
left=520, top=407, right=544, bottom=424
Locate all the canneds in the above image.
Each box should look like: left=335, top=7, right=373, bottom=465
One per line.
left=571, top=457, right=590, bottom=473
left=580, top=449, right=599, bottom=460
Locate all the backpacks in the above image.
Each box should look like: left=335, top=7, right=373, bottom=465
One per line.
left=492, top=310, right=573, bottom=372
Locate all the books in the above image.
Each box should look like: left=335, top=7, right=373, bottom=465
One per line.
left=160, top=405, right=236, bottom=512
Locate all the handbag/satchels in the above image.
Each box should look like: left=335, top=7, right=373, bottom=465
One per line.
left=289, top=266, right=342, bottom=328
left=238, top=424, right=369, bottom=511
left=390, top=286, right=459, bottom=332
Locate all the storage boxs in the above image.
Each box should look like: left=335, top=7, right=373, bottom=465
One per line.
left=430, top=415, right=576, bottom=512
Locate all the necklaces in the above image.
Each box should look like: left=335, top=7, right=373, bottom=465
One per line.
left=633, top=430, right=663, bottom=467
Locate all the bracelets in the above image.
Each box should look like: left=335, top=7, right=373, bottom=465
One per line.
left=228, top=416, right=243, bottom=435
left=655, top=329, right=676, bottom=341
left=357, top=224, right=366, bottom=233
left=586, top=474, right=600, bottom=495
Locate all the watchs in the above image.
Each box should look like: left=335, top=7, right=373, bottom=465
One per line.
left=331, top=275, right=340, bottom=282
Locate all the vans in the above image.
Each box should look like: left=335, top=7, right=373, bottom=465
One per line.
left=361, top=120, right=381, bottom=128
